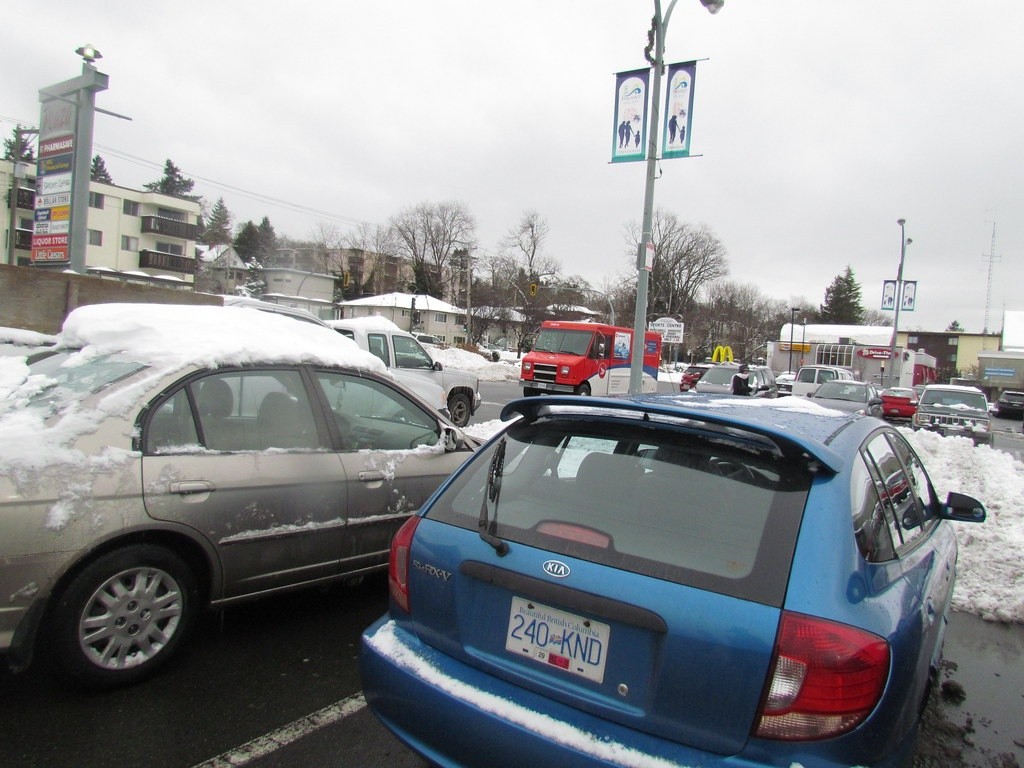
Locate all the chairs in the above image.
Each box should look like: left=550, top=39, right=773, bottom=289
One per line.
left=573, top=450, right=639, bottom=518
left=246, top=391, right=316, bottom=448
left=930, top=396, right=943, bottom=404
left=640, top=448, right=729, bottom=516
left=188, top=379, right=254, bottom=450
left=370, top=346, right=384, bottom=361
left=961, top=398, right=976, bottom=407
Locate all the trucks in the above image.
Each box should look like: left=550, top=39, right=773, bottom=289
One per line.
left=519, top=319, right=664, bottom=399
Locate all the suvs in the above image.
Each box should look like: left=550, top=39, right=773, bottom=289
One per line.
left=909, top=382, right=1000, bottom=447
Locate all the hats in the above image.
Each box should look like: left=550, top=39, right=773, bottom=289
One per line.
left=743, top=366, right=749, bottom=370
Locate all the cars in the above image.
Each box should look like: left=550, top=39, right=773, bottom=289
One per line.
left=992, top=389, right=1024, bottom=419
left=357, top=393, right=989, bottom=768
left=678, top=360, right=927, bottom=423
left=0, top=299, right=526, bottom=695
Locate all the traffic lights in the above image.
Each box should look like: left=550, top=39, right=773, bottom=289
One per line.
left=413, top=310, right=421, bottom=325
left=529, top=283, right=538, bottom=297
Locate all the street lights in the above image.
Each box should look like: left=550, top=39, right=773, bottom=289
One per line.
left=798, top=323, right=806, bottom=366
left=788, top=306, right=801, bottom=375
left=888, top=217, right=914, bottom=388
left=628, top=0, right=727, bottom=395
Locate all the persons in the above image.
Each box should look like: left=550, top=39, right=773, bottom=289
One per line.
left=729, top=365, right=752, bottom=395
